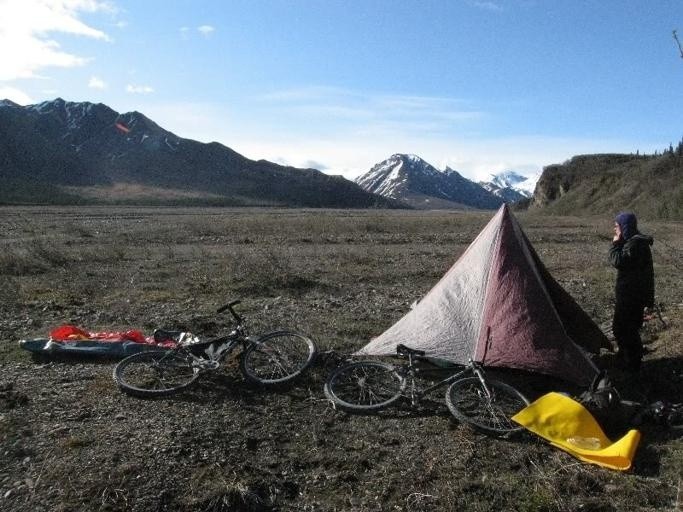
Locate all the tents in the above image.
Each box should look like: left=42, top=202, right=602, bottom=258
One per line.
left=349, top=200, right=619, bottom=399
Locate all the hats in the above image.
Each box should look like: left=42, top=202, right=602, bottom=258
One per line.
left=614, top=213, right=639, bottom=239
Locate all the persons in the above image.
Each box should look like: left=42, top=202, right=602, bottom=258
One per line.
left=603, top=211, right=656, bottom=371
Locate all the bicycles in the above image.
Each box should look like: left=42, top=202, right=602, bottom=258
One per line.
left=111, top=299, right=318, bottom=400
left=326, top=331, right=531, bottom=438
